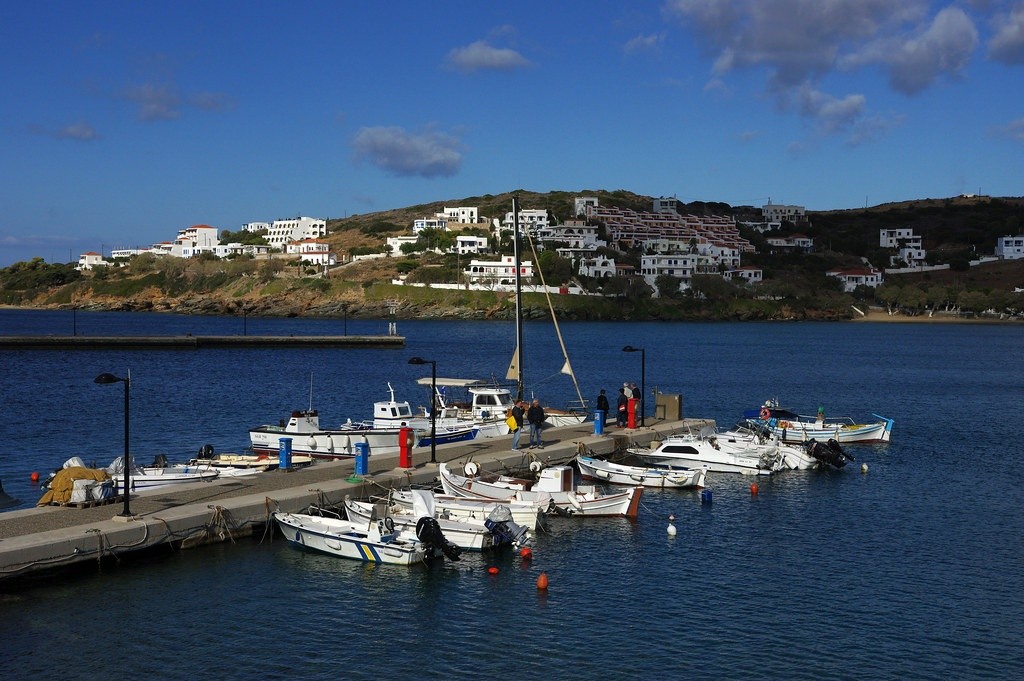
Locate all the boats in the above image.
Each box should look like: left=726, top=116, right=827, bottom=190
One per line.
left=247, top=409, right=429, bottom=460
left=109, top=443, right=314, bottom=477
left=621, top=345, right=645, bottom=427
left=275, top=511, right=461, bottom=566
left=389, top=488, right=574, bottom=532
left=438, top=463, right=645, bottom=517
left=93, top=374, right=132, bottom=518
left=492, top=474, right=599, bottom=495
left=407, top=357, right=438, bottom=463
left=42, top=457, right=220, bottom=493
left=626, top=419, right=856, bottom=476
left=344, top=491, right=522, bottom=553
left=339, top=401, right=480, bottom=446
left=734, top=400, right=895, bottom=443
left=575, top=443, right=709, bottom=486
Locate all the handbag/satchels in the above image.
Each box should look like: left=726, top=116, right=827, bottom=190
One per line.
left=505, top=415, right=518, bottom=431
left=619, top=404, right=626, bottom=411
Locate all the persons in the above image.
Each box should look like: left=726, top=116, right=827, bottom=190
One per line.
left=631, top=383, right=641, bottom=399
left=597, top=389, right=610, bottom=427
left=528, top=399, right=544, bottom=449
left=760, top=406, right=770, bottom=418
left=512, top=400, right=525, bottom=451
left=614, top=388, right=628, bottom=428
left=623, top=382, right=633, bottom=399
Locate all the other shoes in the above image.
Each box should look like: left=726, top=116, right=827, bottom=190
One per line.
left=620, top=425, right=625, bottom=427
left=529, top=445, right=533, bottom=448
left=512, top=449, right=519, bottom=451
left=538, top=445, right=544, bottom=448
left=615, top=425, right=621, bottom=428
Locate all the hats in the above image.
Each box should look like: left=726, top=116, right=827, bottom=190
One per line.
left=617, top=388, right=625, bottom=393
left=624, top=382, right=629, bottom=386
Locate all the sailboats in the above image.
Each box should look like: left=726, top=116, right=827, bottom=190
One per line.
left=413, top=193, right=589, bottom=431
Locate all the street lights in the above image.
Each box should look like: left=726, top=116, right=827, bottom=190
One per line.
left=73, top=307, right=77, bottom=336
left=344, top=306, right=347, bottom=336
left=244, top=309, right=247, bottom=334
